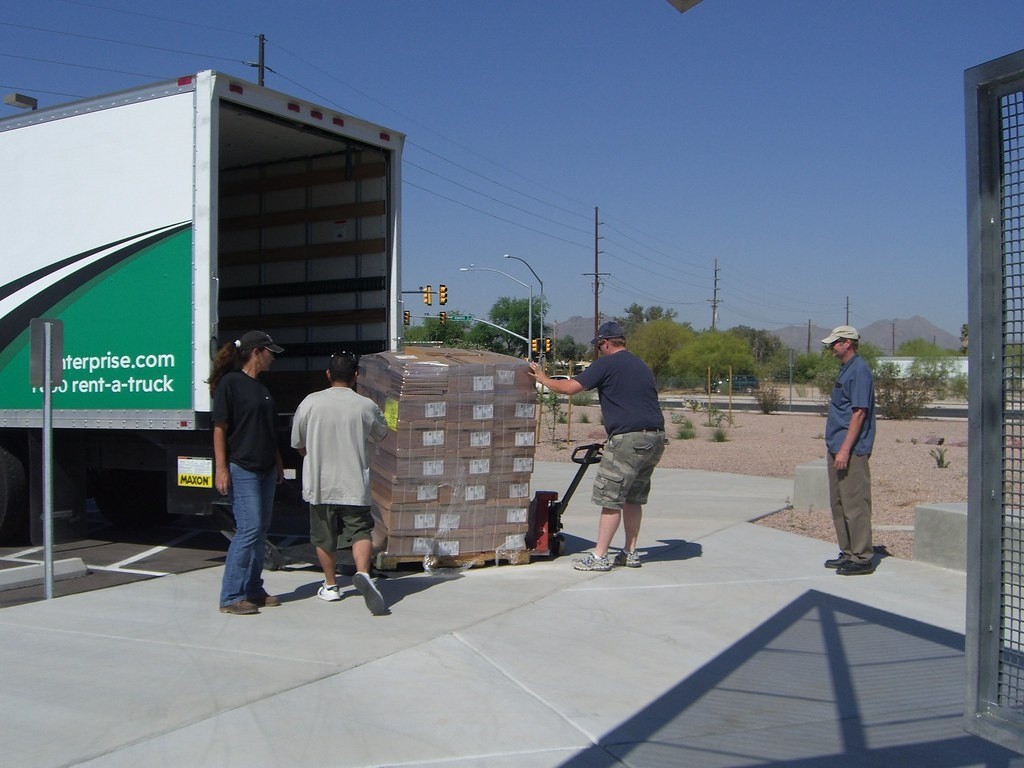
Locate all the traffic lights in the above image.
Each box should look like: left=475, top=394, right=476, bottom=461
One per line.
left=439, top=285, right=448, bottom=305
left=423, top=285, right=432, bottom=306
left=532, top=339, right=538, bottom=352
left=403, top=311, right=410, bottom=325
left=545, top=338, right=552, bottom=353
left=440, top=311, right=446, bottom=326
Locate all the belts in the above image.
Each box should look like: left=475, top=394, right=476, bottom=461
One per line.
left=633, top=427, right=659, bottom=432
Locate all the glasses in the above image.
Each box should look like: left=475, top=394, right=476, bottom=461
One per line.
left=596, top=339, right=608, bottom=351
left=327, top=350, right=357, bottom=372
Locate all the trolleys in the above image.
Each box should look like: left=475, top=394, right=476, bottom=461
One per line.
left=525, top=442, right=605, bottom=561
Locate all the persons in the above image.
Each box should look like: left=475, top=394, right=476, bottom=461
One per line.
left=291, top=351, right=389, bottom=616
left=821, top=326, right=876, bottom=576
left=527, top=321, right=665, bottom=571
left=206, top=331, right=284, bottom=615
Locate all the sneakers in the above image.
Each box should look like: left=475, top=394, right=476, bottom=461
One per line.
left=219, top=600, right=259, bottom=615
left=572, top=554, right=610, bottom=571
left=607, top=549, right=643, bottom=567
left=246, top=593, right=280, bottom=608
left=317, top=581, right=340, bottom=602
left=352, top=570, right=386, bottom=615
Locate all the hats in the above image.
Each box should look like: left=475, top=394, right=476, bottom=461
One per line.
left=588, top=320, right=625, bottom=343
left=821, top=325, right=859, bottom=344
left=238, top=330, right=285, bottom=353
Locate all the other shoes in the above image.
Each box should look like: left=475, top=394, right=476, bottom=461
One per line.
left=835, top=561, right=874, bottom=575
left=824, top=552, right=852, bottom=569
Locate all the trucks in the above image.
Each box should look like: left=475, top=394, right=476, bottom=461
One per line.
left=0, top=68, right=406, bottom=546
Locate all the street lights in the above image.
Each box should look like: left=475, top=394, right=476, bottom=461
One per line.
left=459, top=267, right=532, bottom=361
left=504, top=255, right=543, bottom=353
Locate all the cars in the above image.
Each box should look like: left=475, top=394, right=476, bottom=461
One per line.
left=536, top=375, right=571, bottom=394
left=705, top=374, right=760, bottom=394
